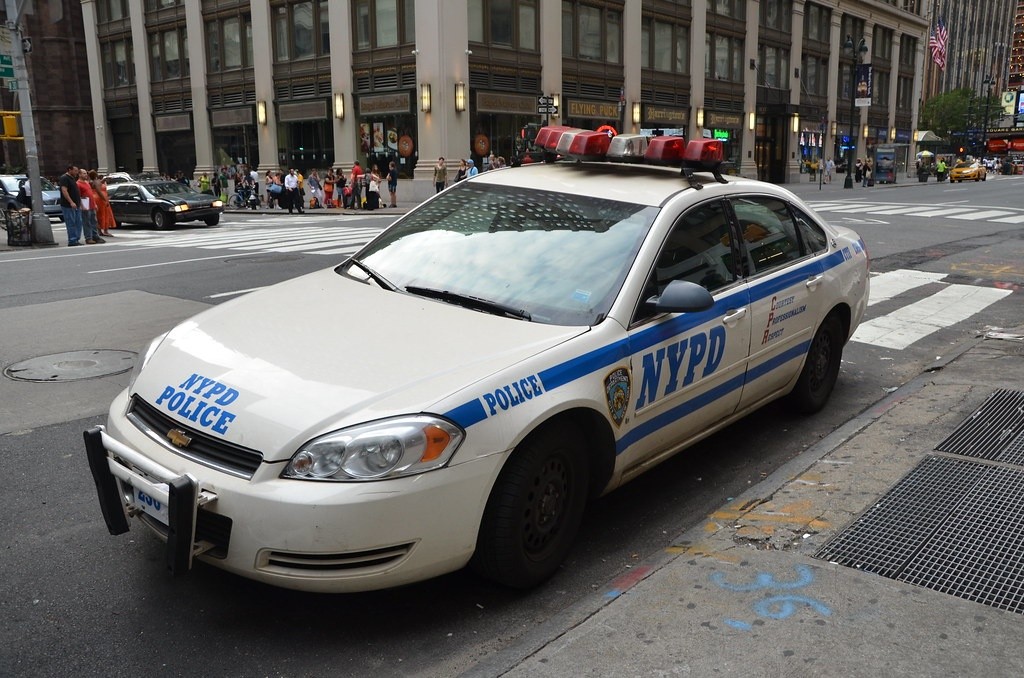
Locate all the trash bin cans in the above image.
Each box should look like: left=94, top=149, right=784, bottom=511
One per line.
left=4, top=210, right=33, bottom=246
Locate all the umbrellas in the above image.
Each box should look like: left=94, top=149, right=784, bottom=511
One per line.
left=917, top=150, right=933, bottom=165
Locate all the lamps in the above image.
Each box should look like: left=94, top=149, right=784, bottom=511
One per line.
left=913, top=129, right=918, bottom=142
left=420, top=83, right=431, bottom=113
left=257, top=99, right=267, bottom=128
left=551, top=93, right=560, bottom=119
left=791, top=113, right=799, bottom=133
left=832, top=121, right=837, bottom=135
left=632, top=102, right=641, bottom=124
left=890, top=127, right=896, bottom=140
left=863, top=124, right=868, bottom=139
left=455, top=82, right=466, bottom=112
left=749, top=112, right=755, bottom=131
left=334, top=93, right=344, bottom=120
left=696, top=106, right=704, bottom=128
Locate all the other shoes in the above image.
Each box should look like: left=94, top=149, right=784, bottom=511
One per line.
left=68, top=242, right=84, bottom=246
left=99, top=233, right=114, bottom=237
left=299, top=210, right=305, bottom=214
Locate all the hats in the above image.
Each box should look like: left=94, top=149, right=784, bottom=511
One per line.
left=466, top=159, right=473, bottom=164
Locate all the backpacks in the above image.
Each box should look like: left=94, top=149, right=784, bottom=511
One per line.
left=309, top=197, right=319, bottom=209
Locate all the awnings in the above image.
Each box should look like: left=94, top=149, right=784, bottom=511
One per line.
left=986, top=139, right=1024, bottom=153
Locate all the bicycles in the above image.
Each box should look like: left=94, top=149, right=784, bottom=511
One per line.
left=229, top=189, right=258, bottom=210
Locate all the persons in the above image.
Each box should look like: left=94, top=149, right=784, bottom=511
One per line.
left=916, top=157, right=1024, bottom=182
left=161, top=148, right=534, bottom=210
left=19, top=165, right=116, bottom=246
left=800, top=157, right=874, bottom=187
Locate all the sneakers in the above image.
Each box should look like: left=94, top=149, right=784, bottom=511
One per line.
left=86, top=238, right=107, bottom=244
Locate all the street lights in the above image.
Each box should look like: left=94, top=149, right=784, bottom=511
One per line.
left=980, top=74, right=996, bottom=165
left=843, top=33, right=868, bottom=188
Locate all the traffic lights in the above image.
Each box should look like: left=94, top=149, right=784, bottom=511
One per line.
left=521, top=127, right=529, bottom=141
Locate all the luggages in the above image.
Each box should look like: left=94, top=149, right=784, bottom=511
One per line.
left=389, top=204, right=397, bottom=207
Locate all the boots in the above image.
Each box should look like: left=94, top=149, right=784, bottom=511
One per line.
left=273, top=199, right=282, bottom=210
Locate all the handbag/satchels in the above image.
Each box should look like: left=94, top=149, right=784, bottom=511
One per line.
left=454, top=173, right=459, bottom=182
left=319, top=184, right=322, bottom=190
left=270, top=183, right=282, bottom=194
left=301, top=189, right=305, bottom=196
left=324, top=183, right=333, bottom=193
left=265, top=184, right=272, bottom=190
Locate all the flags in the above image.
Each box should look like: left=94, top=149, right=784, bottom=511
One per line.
left=929, top=18, right=948, bottom=71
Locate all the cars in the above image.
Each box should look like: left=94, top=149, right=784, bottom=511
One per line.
left=0, top=174, right=65, bottom=223
left=82, top=126, right=870, bottom=596
left=950, top=161, right=988, bottom=183
left=101, top=171, right=225, bottom=231
